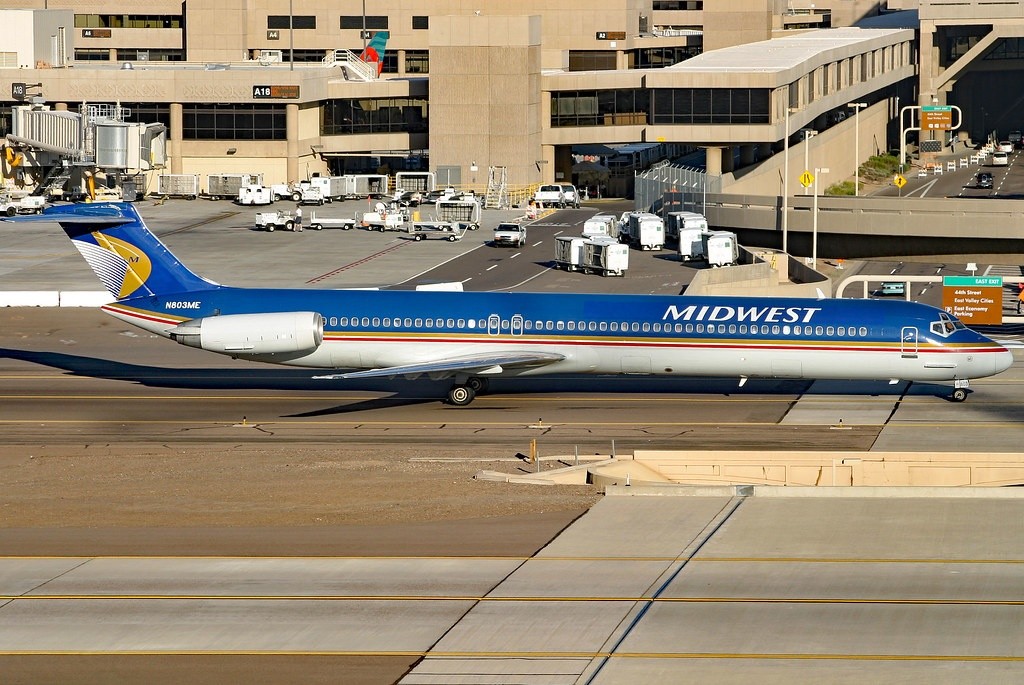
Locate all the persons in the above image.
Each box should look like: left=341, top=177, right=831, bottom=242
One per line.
left=293, top=204, right=303, bottom=232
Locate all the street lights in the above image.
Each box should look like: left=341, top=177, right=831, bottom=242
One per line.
left=804, top=131, right=817, bottom=194
left=813, top=168, right=829, bottom=271
left=783, top=107, right=797, bottom=254
left=848, top=103, right=867, bottom=197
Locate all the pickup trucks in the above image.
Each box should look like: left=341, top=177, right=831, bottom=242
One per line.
left=493, top=221, right=526, bottom=247
left=535, top=184, right=581, bottom=209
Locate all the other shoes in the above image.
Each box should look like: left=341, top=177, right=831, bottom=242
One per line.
left=293, top=229, right=295, bottom=232
left=298, top=230, right=302, bottom=232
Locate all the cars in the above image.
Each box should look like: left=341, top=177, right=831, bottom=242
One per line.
left=975, top=172, right=995, bottom=189
left=0, top=202, right=1013, bottom=406
left=881, top=283, right=904, bottom=296
left=997, top=130, right=1024, bottom=154
left=992, top=152, right=1008, bottom=166
